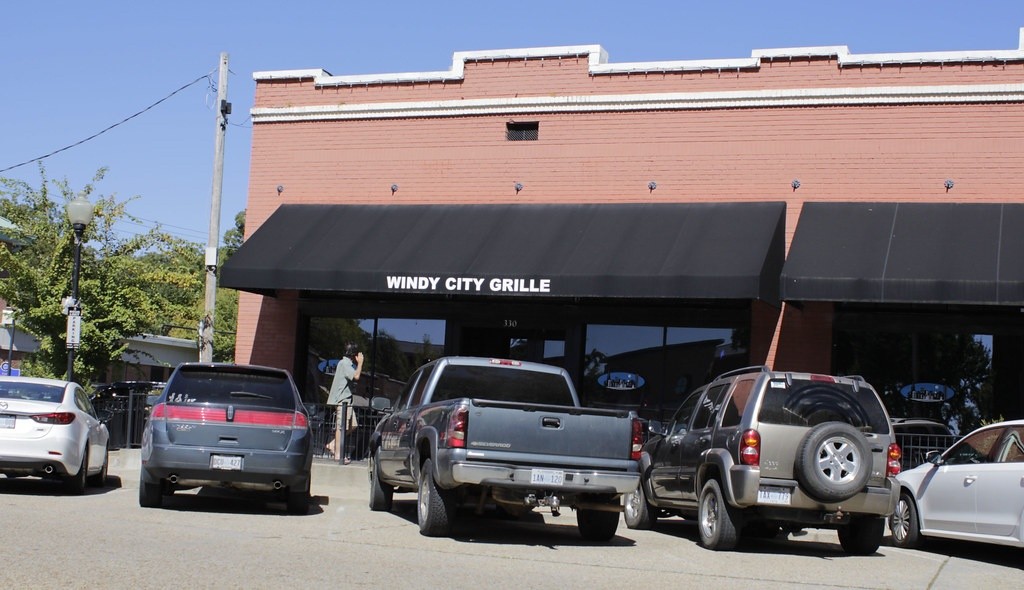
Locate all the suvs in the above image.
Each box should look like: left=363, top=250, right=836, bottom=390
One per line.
left=138, top=361, right=315, bottom=517
left=623, top=364, right=902, bottom=557
left=885, top=415, right=955, bottom=466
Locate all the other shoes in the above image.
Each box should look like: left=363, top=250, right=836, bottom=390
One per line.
left=326, top=443, right=334, bottom=456
left=335, top=457, right=350, bottom=463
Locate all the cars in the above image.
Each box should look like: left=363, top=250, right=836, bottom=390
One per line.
left=882, top=419, right=1024, bottom=550
left=0, top=375, right=111, bottom=495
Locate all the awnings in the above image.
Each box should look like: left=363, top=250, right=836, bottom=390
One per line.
left=216, top=201, right=785, bottom=306
left=779, top=202, right=1024, bottom=310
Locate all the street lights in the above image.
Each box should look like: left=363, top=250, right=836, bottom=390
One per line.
left=66, top=190, right=94, bottom=382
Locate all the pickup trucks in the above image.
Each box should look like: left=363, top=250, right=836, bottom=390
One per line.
left=366, top=355, right=645, bottom=538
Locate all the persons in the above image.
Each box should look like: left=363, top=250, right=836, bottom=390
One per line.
left=326, top=340, right=364, bottom=464
